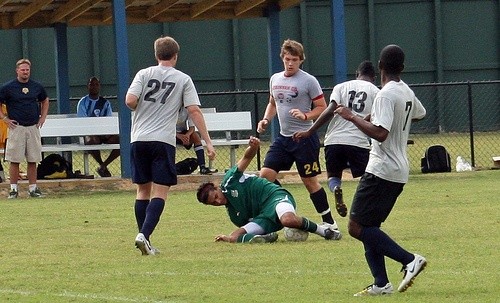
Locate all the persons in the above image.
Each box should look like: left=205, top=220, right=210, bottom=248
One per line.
left=293, top=60, right=380, bottom=216
left=176, top=104, right=212, bottom=175
left=334, top=45, right=427, bottom=296
left=125, top=36, right=216, bottom=256
left=254, top=39, right=339, bottom=231
left=76, top=76, right=120, bottom=178
left=196, top=136, right=343, bottom=241
left=0, top=59, right=50, bottom=199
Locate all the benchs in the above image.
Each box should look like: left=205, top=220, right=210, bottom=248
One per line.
left=39, top=107, right=255, bottom=174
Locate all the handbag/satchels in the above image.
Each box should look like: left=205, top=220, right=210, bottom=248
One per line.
left=38, top=153, right=71, bottom=176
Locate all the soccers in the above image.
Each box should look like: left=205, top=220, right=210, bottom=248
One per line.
left=284, top=228, right=308, bottom=241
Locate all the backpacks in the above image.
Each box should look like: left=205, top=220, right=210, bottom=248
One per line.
left=175, top=157, right=198, bottom=174
left=421, top=144, right=452, bottom=173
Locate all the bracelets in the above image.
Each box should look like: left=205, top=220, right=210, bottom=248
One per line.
left=2, top=116, right=7, bottom=121
left=304, top=114, right=307, bottom=121
left=264, top=118, right=269, bottom=123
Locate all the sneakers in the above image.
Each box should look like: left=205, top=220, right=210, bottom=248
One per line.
left=354, top=282, right=395, bottom=298
left=283, top=228, right=308, bottom=240
left=333, top=186, right=347, bottom=218
left=398, top=253, right=427, bottom=293
left=135, top=233, right=160, bottom=255
left=319, top=220, right=338, bottom=230
left=321, top=228, right=341, bottom=240
left=253, top=231, right=278, bottom=243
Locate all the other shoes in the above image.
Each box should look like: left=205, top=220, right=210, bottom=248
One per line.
left=97, top=166, right=110, bottom=177
left=8, top=189, right=18, bottom=199
left=28, top=189, right=41, bottom=198
left=201, top=166, right=212, bottom=175
